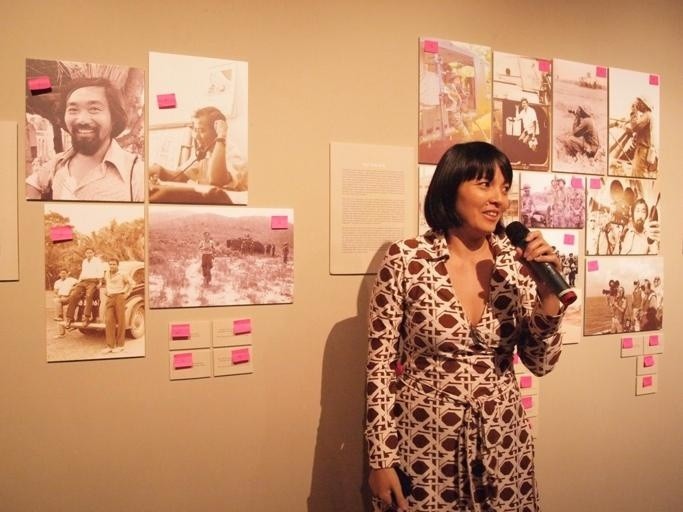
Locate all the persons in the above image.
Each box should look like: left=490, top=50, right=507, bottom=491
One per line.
left=616, top=96, right=655, bottom=177
left=586, top=179, right=660, bottom=254
left=51, top=268, right=78, bottom=338
left=25, top=77, right=144, bottom=204
left=562, top=103, right=601, bottom=164
left=357, top=141, right=570, bottom=512
left=514, top=96, right=539, bottom=163
left=239, top=238, right=289, bottom=264
left=601, top=276, right=663, bottom=333
left=439, top=61, right=474, bottom=141
left=148, top=106, right=248, bottom=193
left=197, top=231, right=216, bottom=288
left=520, top=177, right=585, bottom=230
left=61, top=248, right=107, bottom=330
left=100, top=257, right=133, bottom=354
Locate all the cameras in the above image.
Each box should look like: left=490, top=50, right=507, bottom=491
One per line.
left=568, top=109, right=578, bottom=115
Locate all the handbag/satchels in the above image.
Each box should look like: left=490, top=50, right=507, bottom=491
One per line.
left=527, top=138, right=539, bottom=152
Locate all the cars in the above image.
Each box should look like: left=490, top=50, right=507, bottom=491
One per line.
left=62, top=260, right=145, bottom=340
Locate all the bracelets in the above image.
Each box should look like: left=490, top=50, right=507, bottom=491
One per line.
left=215, top=138, right=224, bottom=143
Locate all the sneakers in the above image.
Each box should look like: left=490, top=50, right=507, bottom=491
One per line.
left=100, top=347, right=124, bottom=352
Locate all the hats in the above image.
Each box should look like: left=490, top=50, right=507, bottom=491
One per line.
left=636, top=94, right=653, bottom=112
left=579, top=104, right=594, bottom=117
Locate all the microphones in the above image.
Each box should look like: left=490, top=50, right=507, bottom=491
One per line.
left=503, top=222, right=577, bottom=306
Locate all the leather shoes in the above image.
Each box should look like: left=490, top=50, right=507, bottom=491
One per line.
left=53, top=316, right=91, bottom=340
